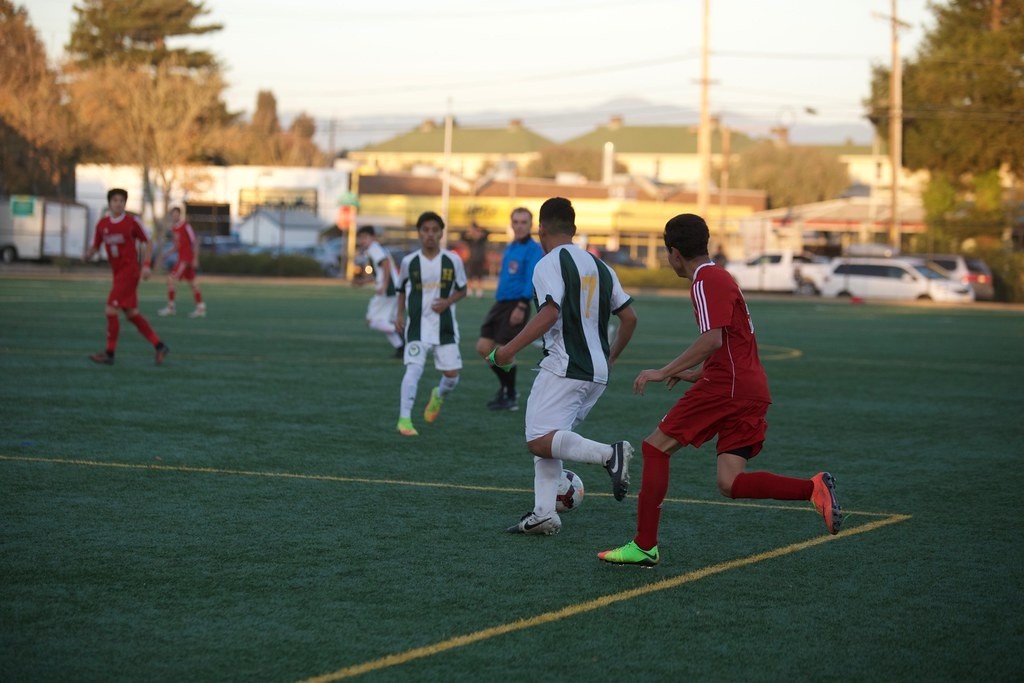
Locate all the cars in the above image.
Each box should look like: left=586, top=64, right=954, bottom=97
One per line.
left=724, top=250, right=814, bottom=294
left=798, top=244, right=995, bottom=304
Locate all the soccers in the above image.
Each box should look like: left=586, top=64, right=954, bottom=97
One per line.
left=554, top=468, right=584, bottom=512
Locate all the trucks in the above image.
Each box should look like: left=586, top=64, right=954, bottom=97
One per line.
left=1, top=195, right=88, bottom=263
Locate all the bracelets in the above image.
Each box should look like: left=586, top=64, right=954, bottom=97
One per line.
left=144, top=262, right=151, bottom=267
left=516, top=305, right=527, bottom=311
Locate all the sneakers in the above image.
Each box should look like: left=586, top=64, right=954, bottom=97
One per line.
left=396, top=418, right=419, bottom=436
left=604, top=440, right=635, bottom=502
left=597, top=540, right=658, bottom=569
left=156, top=346, right=170, bottom=365
left=91, top=351, right=113, bottom=365
left=506, top=511, right=562, bottom=535
left=424, top=387, right=444, bottom=423
left=809, top=470, right=841, bottom=535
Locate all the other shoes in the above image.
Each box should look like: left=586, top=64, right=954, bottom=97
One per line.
left=160, top=302, right=177, bottom=316
left=487, top=396, right=507, bottom=409
left=190, top=304, right=206, bottom=318
left=394, top=344, right=405, bottom=359
left=508, top=393, right=518, bottom=411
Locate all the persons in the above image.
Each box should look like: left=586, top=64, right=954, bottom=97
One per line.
left=597, top=214, right=842, bottom=567
left=158, top=208, right=208, bottom=322
left=711, top=244, right=728, bottom=269
left=485, top=198, right=638, bottom=535
left=83, top=188, right=170, bottom=366
left=351, top=225, right=405, bottom=359
left=476, top=208, right=544, bottom=413
left=395, top=211, right=467, bottom=436
